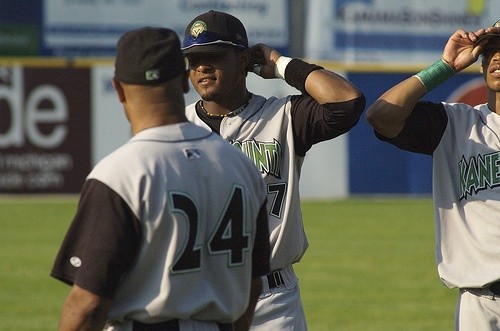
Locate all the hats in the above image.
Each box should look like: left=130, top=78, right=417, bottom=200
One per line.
left=180, top=10, right=248, bottom=54
left=115, top=26, right=185, bottom=84
left=473, top=20, right=500, bottom=49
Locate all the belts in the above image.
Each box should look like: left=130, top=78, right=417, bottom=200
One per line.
left=133, top=319, right=234, bottom=331
left=267, top=271, right=284, bottom=289
left=490, top=283, right=500, bottom=297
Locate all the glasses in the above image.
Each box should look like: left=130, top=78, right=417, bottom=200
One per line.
left=181, top=31, right=245, bottom=50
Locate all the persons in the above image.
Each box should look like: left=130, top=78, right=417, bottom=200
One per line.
left=180, top=10, right=365, bottom=331
left=365, top=21, right=500, bottom=331
left=49, top=27, right=272, bottom=331
